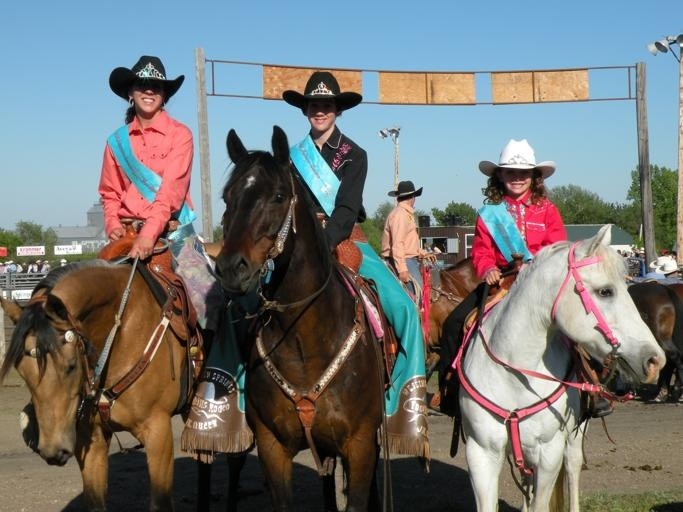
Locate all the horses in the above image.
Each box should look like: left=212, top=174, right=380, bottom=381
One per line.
left=210, top=125, right=407, bottom=511
left=0, top=248, right=246, bottom=512
left=423, top=249, right=482, bottom=411
left=447, top=223, right=666, bottom=512
left=628, top=278, right=682, bottom=405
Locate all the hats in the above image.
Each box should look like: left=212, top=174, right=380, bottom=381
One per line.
left=650, top=256, right=683, bottom=274
left=109, top=56, right=185, bottom=98
left=479, top=139, right=556, bottom=178
left=283, top=72, right=361, bottom=111
left=388, top=181, right=422, bottom=197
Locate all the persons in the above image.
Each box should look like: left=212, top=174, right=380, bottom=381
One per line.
left=0, top=257, right=75, bottom=275
left=616, top=246, right=679, bottom=283
left=376, top=176, right=427, bottom=295
left=97, top=54, right=217, bottom=317
left=278, top=71, right=432, bottom=408
left=430, top=137, right=573, bottom=415
